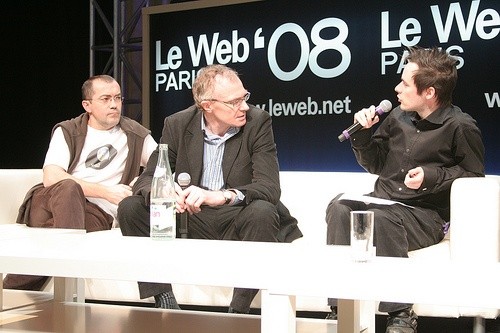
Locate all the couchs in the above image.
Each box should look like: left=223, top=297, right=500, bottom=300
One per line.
left=0, top=166, right=500, bottom=333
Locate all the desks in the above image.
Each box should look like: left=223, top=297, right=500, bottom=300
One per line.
left=0, top=223, right=474, bottom=333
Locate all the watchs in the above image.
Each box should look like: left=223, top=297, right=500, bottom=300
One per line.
left=220, top=189, right=232, bottom=206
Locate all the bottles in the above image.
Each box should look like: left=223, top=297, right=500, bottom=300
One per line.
left=150, top=143, right=176, bottom=240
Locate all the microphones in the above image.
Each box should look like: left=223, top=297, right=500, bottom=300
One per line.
left=177, top=172, right=191, bottom=239
left=338, top=100, right=392, bottom=143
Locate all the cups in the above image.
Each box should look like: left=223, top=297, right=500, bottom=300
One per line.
left=350, top=211, right=375, bottom=262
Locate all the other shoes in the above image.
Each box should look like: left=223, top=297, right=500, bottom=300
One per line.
left=386, top=310, right=418, bottom=333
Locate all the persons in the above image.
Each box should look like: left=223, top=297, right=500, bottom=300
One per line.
left=324, top=48, right=487, bottom=333
left=116, top=67, right=303, bottom=314
left=4, top=75, right=159, bottom=291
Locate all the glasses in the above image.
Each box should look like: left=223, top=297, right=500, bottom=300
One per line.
left=89, top=96, right=124, bottom=104
left=202, top=91, right=250, bottom=108
